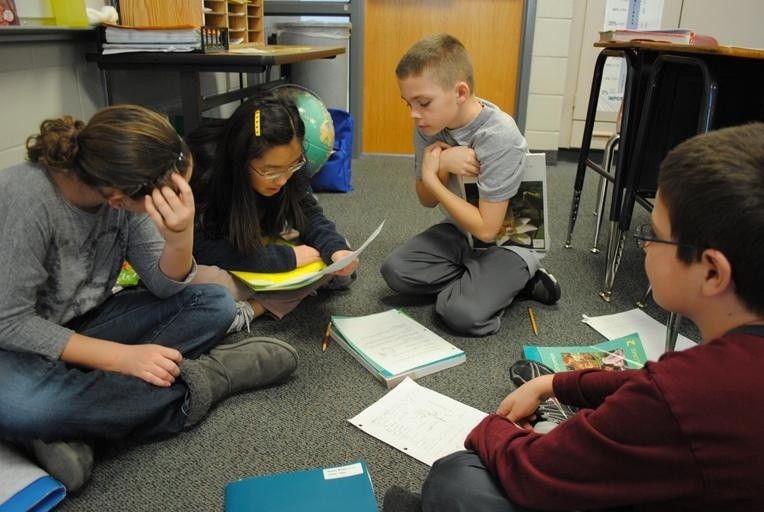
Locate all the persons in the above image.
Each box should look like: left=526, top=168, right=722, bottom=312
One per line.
left=0, top=103, right=299, bottom=495
left=182, top=92, right=360, bottom=335
left=422, top=119, right=764, bottom=512
left=379, top=33, right=562, bottom=338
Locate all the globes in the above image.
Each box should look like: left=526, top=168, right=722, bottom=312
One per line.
left=268, top=83, right=335, bottom=178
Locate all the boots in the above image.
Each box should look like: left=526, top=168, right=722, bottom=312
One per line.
left=174, top=336, right=301, bottom=427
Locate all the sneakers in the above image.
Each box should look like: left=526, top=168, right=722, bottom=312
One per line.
left=383, top=485, right=423, bottom=512
left=516, top=267, right=562, bottom=306
left=20, top=438, right=95, bottom=494
left=225, top=300, right=256, bottom=335
left=318, top=269, right=357, bottom=290
left=509, top=359, right=585, bottom=429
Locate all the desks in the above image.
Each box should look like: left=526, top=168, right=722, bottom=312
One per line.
left=563, top=40, right=764, bottom=309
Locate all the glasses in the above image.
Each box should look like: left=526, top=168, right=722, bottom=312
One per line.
left=632, top=222, right=728, bottom=252
left=246, top=150, right=307, bottom=181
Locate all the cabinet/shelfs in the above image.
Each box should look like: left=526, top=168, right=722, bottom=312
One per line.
left=85, top=0, right=345, bottom=137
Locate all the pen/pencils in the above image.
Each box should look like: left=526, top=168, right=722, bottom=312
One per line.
left=528, top=306, right=539, bottom=336
left=322, top=321, right=332, bottom=352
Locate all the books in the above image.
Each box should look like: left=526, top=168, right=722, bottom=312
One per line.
left=598, top=28, right=692, bottom=44
left=228, top=234, right=327, bottom=293
left=438, top=152, right=551, bottom=252
left=523, top=332, right=649, bottom=373
left=223, top=460, right=379, bottom=512
left=101, top=25, right=220, bottom=56
left=328, top=306, right=466, bottom=389
